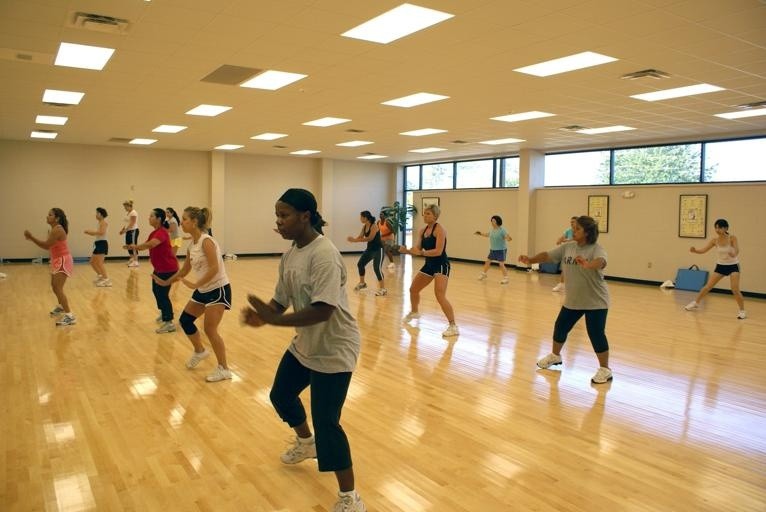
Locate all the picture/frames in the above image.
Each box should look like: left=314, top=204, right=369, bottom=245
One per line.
left=588, top=194, right=609, bottom=233
left=422, top=197, right=439, bottom=216
left=678, top=193, right=708, bottom=238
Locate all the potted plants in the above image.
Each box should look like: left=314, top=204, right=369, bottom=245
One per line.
left=380, top=201, right=417, bottom=256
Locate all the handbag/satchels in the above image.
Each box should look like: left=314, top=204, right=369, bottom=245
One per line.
left=674, top=264, right=709, bottom=292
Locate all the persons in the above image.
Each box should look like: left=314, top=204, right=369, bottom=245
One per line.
left=345, top=210, right=389, bottom=296
left=151, top=205, right=232, bottom=381
left=181, top=230, right=210, bottom=241
left=504, top=364, right=608, bottom=508
left=82, top=207, right=113, bottom=288
left=238, top=185, right=365, bottom=512
left=395, top=203, right=462, bottom=338
left=403, top=320, right=457, bottom=447
left=472, top=214, right=513, bottom=284
left=118, top=199, right=140, bottom=268
left=481, top=278, right=511, bottom=355
left=122, top=206, right=181, bottom=335
left=166, top=207, right=181, bottom=260
left=53, top=326, right=77, bottom=391
left=376, top=211, right=397, bottom=269
left=682, top=217, right=747, bottom=320
left=519, top=215, right=614, bottom=386
left=152, top=328, right=176, bottom=417
left=551, top=216, right=580, bottom=292
left=686, top=314, right=743, bottom=408
left=122, top=268, right=141, bottom=326
left=352, top=290, right=391, bottom=370
left=90, top=288, right=115, bottom=342
left=23, top=208, right=76, bottom=326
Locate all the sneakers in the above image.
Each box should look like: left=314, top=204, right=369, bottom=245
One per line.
left=374, top=287, right=387, bottom=296
left=682, top=301, right=700, bottom=311
left=354, top=282, right=368, bottom=291
left=536, top=353, right=562, bottom=370
left=280, top=436, right=318, bottom=465
left=126, top=258, right=140, bottom=267
left=478, top=272, right=488, bottom=281
left=332, top=489, right=368, bottom=512
left=93, top=274, right=113, bottom=287
left=155, top=312, right=176, bottom=334
left=400, top=311, right=421, bottom=323
left=442, top=324, right=459, bottom=337
left=386, top=262, right=396, bottom=268
left=500, top=277, right=509, bottom=285
left=590, top=365, right=613, bottom=384
left=736, top=310, right=747, bottom=319
left=185, top=349, right=209, bottom=370
left=206, top=363, right=232, bottom=382
left=552, top=284, right=564, bottom=292
left=48, top=305, right=77, bottom=327
